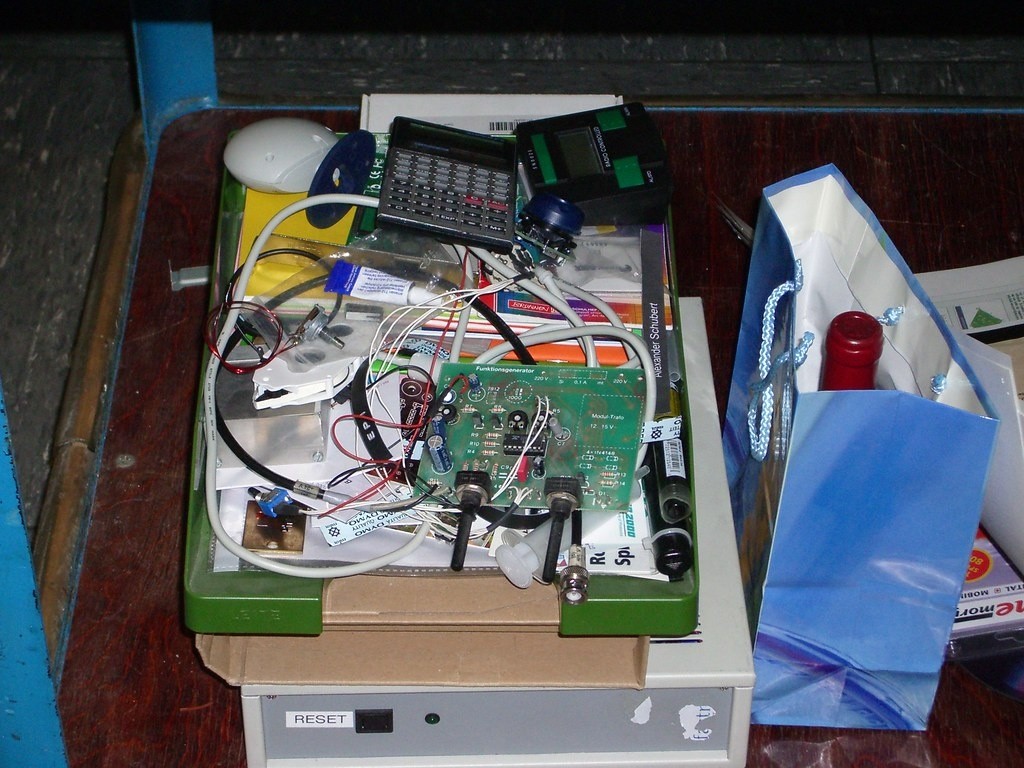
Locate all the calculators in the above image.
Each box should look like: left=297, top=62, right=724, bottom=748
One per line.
left=376, top=116, right=519, bottom=256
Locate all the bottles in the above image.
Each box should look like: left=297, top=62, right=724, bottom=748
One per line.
left=820, top=310, right=884, bottom=396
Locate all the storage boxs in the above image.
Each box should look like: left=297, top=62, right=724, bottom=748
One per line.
left=240, top=297, right=757, bottom=768
left=182, top=127, right=701, bottom=636
left=912, top=252, right=1024, bottom=662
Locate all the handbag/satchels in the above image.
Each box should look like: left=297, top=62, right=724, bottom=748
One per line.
left=721, top=162, right=1001, bottom=733
